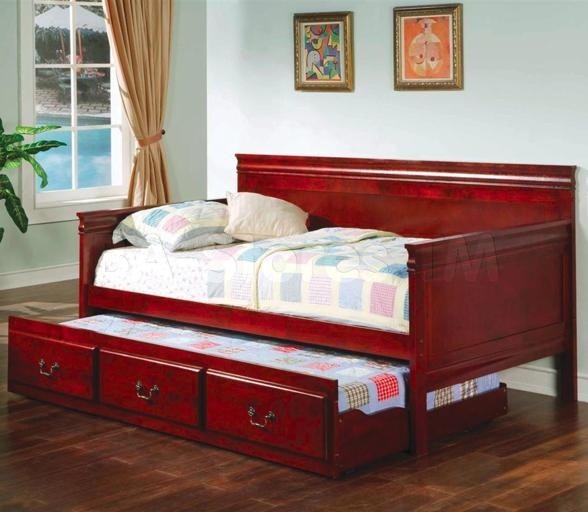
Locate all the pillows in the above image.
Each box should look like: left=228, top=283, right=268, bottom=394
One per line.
left=221, top=190, right=310, bottom=244
left=111, top=199, right=234, bottom=253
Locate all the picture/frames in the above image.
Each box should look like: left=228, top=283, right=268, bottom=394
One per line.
left=393, top=3, right=463, bottom=89
left=295, top=12, right=354, bottom=94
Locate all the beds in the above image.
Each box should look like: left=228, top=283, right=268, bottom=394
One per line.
left=79, top=152, right=577, bottom=456
left=7, top=314, right=509, bottom=479
